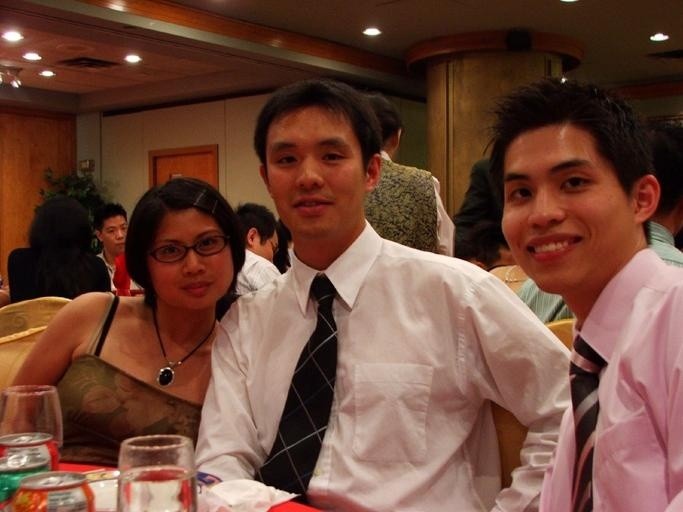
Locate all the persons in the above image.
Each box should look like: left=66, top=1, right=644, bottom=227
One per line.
left=484, top=73, right=683, bottom=512
left=648, top=115, right=683, bottom=246
left=363, top=96, right=455, bottom=256
left=9, top=196, right=111, bottom=304
left=9, top=176, right=246, bottom=465
left=454, top=158, right=519, bottom=270
left=96, top=203, right=145, bottom=291
left=234, top=203, right=290, bottom=295
left=190, top=77, right=573, bottom=512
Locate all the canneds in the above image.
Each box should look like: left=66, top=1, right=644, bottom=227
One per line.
left=12, top=472, right=95, bottom=512
left=0, top=431, right=58, bottom=511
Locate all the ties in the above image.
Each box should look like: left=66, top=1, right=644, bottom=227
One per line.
left=569, top=332, right=608, bottom=512
left=253, top=274, right=338, bottom=503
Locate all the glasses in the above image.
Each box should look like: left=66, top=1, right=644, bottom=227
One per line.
left=144, top=232, right=228, bottom=265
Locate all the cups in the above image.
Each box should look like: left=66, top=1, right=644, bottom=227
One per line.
left=119, top=432, right=199, bottom=511
left=0, top=382, right=64, bottom=458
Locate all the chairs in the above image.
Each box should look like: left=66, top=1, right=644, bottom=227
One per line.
left=491, top=318, right=573, bottom=488
left=1, top=297, right=73, bottom=405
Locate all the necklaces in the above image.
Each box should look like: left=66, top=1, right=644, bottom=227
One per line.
left=147, top=303, right=218, bottom=395
